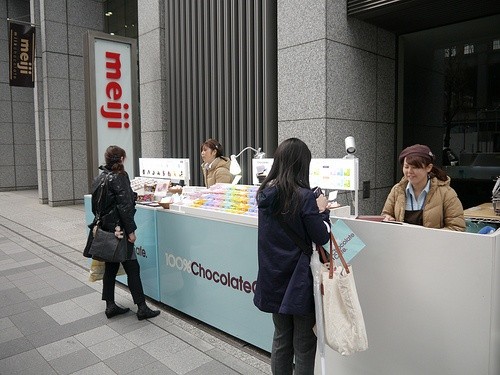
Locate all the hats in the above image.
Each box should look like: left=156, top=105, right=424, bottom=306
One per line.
left=399, top=144, right=433, bottom=165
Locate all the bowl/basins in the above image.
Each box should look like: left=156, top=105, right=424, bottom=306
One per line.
left=168, top=188, right=182, bottom=194
left=157, top=203, right=172, bottom=209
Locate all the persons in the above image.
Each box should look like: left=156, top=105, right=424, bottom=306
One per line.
left=253, top=138, right=331, bottom=375
left=380, top=144, right=466, bottom=232
left=82, top=144, right=160, bottom=321
left=200, top=140, right=233, bottom=189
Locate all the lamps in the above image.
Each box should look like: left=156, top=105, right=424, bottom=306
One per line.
left=342, top=136, right=357, bottom=159
left=229, top=146, right=265, bottom=176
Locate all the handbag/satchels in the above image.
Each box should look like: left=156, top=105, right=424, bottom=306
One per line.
left=322, top=218, right=366, bottom=266
left=88, top=228, right=127, bottom=262
left=88, top=259, right=126, bottom=282
left=309, top=220, right=369, bottom=356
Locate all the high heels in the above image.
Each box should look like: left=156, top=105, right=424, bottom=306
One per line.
left=105, top=306, right=130, bottom=319
left=137, top=306, right=160, bottom=321
